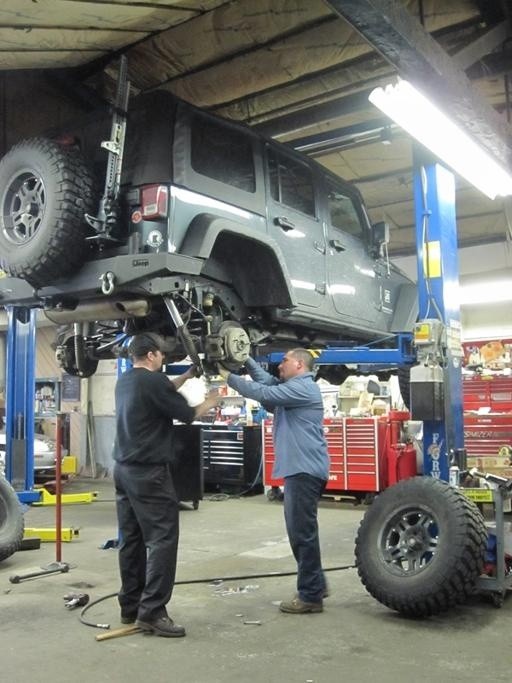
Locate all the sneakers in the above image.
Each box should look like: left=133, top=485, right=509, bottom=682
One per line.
left=120, top=614, right=186, bottom=637
left=279, top=590, right=329, bottom=613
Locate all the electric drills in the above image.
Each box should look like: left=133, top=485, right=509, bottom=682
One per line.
left=64, top=594, right=89, bottom=608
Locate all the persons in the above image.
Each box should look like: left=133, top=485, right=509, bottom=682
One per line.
left=215, top=347, right=332, bottom=615
left=108, top=331, right=226, bottom=636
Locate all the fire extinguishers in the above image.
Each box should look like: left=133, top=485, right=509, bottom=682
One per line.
left=389, top=437, right=416, bottom=486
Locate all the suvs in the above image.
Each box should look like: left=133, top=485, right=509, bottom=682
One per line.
left=2, top=82, right=467, bottom=415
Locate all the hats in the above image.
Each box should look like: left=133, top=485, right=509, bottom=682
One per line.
left=127, top=332, right=177, bottom=356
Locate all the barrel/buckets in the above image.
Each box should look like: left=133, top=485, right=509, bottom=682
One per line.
left=320, top=385, right=343, bottom=420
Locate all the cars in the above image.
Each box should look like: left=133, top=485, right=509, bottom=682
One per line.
left=0, top=419, right=69, bottom=487
